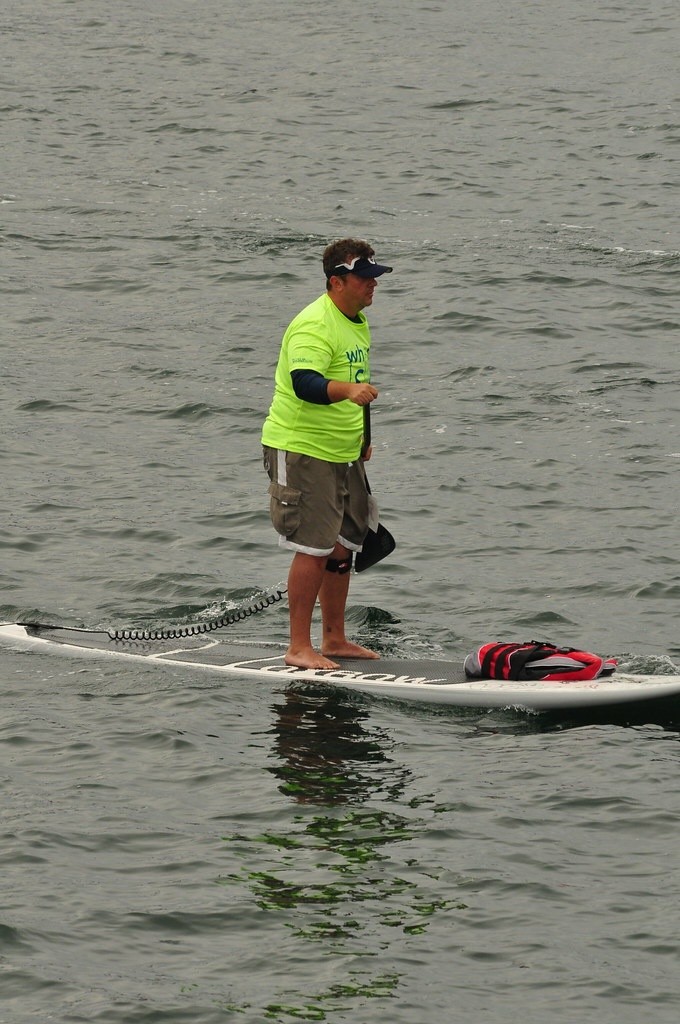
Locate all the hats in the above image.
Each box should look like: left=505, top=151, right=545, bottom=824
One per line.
left=326, top=256, right=393, bottom=280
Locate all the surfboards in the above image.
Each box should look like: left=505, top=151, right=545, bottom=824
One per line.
left=0, top=618, right=680, bottom=706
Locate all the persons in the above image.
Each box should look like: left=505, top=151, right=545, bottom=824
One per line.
left=258, top=236, right=393, bottom=670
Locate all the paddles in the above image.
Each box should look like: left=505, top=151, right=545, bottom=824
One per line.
left=355, top=401, right=396, bottom=574
